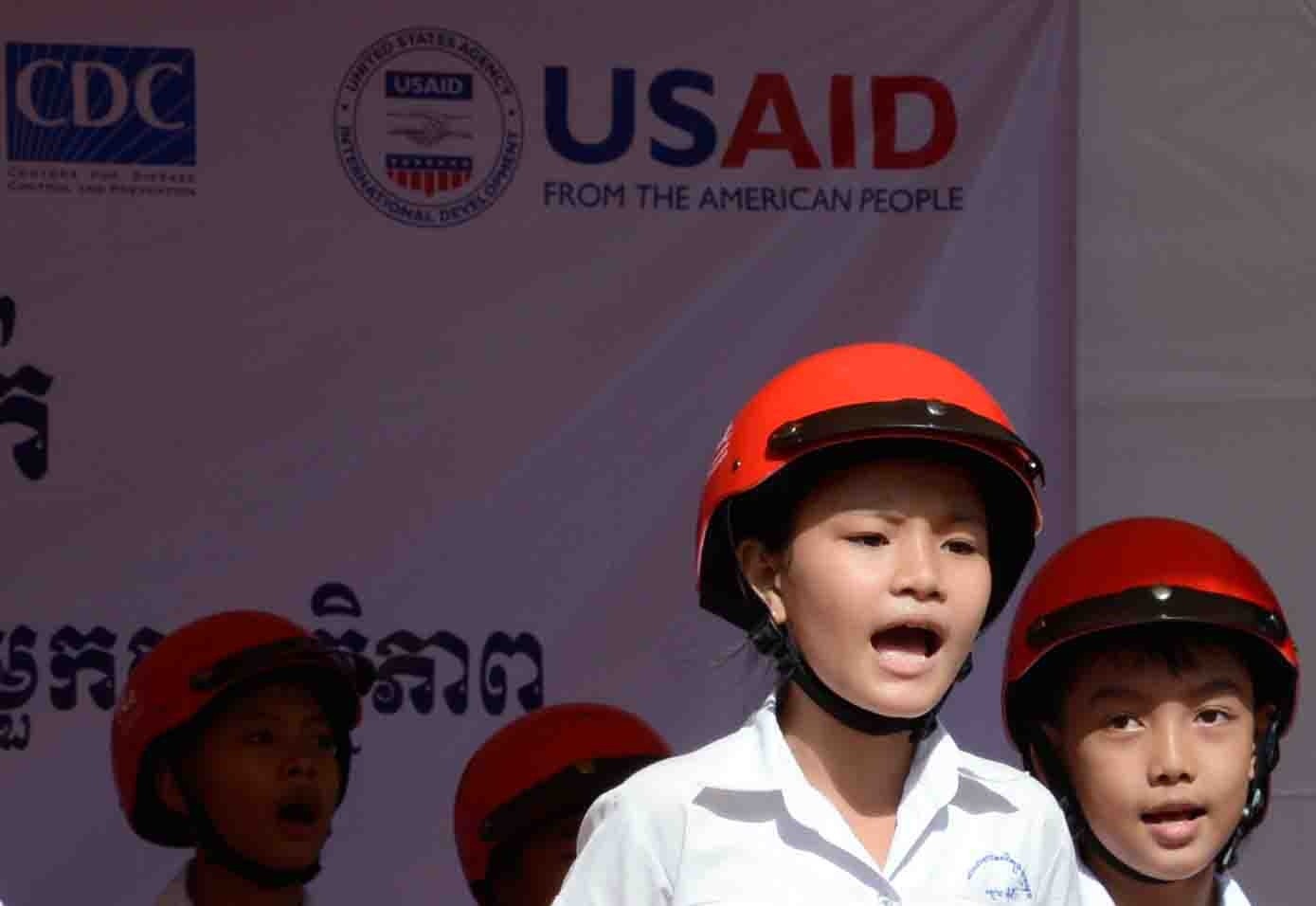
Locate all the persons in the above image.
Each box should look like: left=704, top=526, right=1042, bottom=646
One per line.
left=1001, top=517, right=1306, bottom=906
left=453, top=701, right=673, bottom=906
left=107, top=612, right=363, bottom=906
left=552, top=342, right=1084, bottom=906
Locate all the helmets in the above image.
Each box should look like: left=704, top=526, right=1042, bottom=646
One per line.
left=452, top=701, right=671, bottom=898
left=109, top=609, right=375, bottom=846
left=693, top=341, right=1043, bottom=622
left=1003, top=516, right=1300, bottom=741
